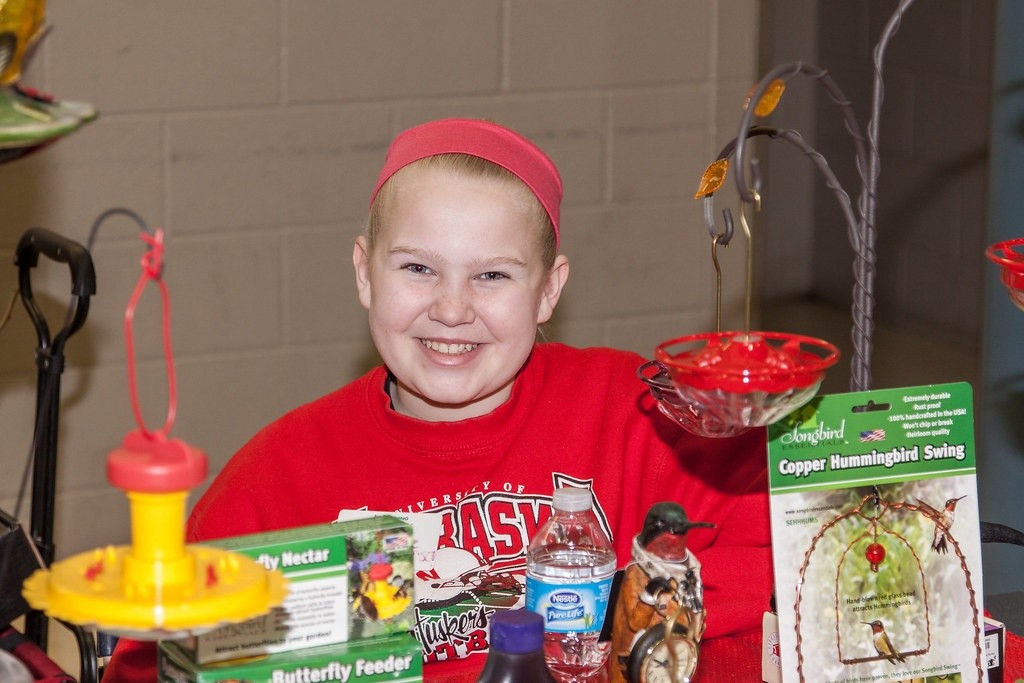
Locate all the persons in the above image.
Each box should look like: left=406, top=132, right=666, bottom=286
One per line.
left=100, top=119, right=774, bottom=683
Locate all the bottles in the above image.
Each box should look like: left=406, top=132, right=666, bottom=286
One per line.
left=478, top=611, right=555, bottom=683
left=526, top=486, right=616, bottom=683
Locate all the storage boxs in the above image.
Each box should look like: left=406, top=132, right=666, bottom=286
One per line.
left=168, top=514, right=416, bottom=667
left=158, top=633, right=424, bottom=683
